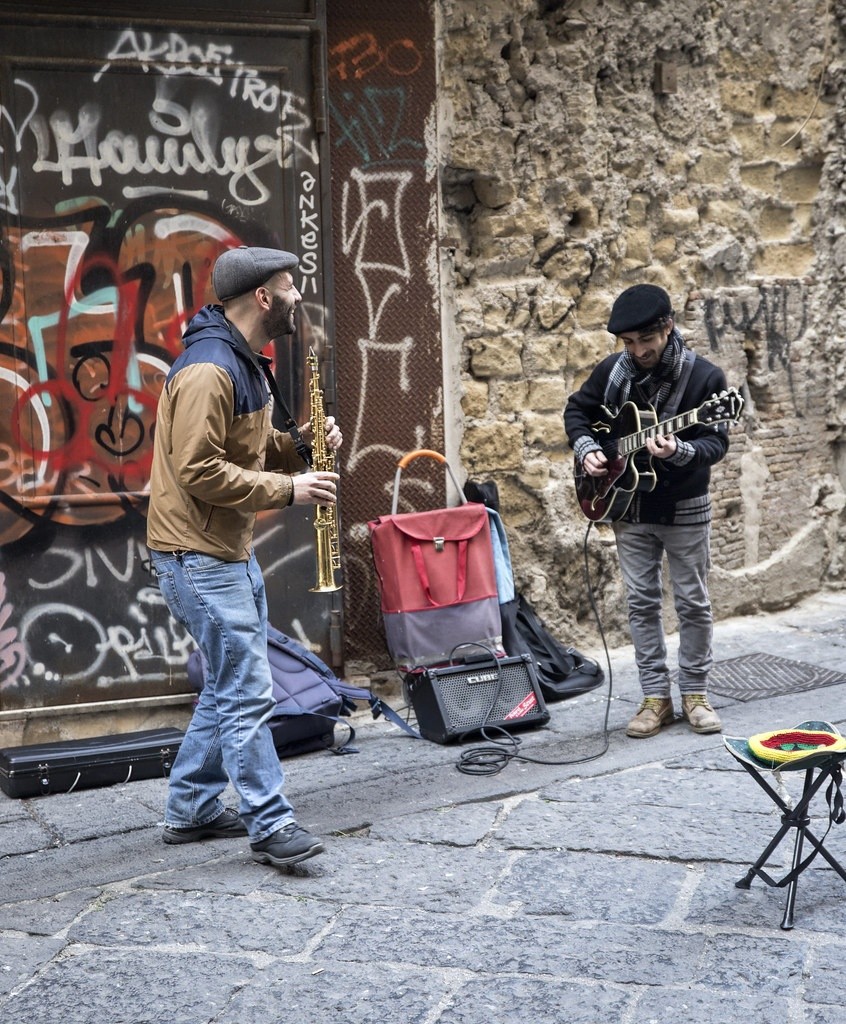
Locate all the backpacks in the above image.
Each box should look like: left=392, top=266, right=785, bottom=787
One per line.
left=503, top=594, right=605, bottom=700
left=186, top=622, right=422, bottom=761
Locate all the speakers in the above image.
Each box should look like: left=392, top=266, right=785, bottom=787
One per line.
left=409, top=653, right=550, bottom=745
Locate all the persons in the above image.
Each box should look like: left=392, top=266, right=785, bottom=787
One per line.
left=563, top=286, right=727, bottom=740
left=145, top=244, right=344, bottom=867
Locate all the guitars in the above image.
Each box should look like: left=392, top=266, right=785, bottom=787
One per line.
left=572, top=385, right=746, bottom=524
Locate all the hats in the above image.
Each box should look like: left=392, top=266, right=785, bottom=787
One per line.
left=212, top=246, right=300, bottom=301
left=607, top=284, right=671, bottom=334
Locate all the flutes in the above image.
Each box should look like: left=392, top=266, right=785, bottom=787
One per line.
left=304, top=343, right=345, bottom=594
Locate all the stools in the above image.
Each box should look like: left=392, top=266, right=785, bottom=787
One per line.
left=721, top=718, right=846, bottom=930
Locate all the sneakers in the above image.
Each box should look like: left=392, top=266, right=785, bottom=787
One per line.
left=162, top=808, right=249, bottom=844
left=250, top=822, right=325, bottom=867
left=681, top=694, right=721, bottom=733
left=625, top=698, right=674, bottom=738
